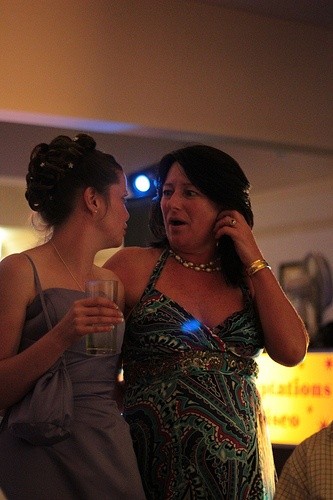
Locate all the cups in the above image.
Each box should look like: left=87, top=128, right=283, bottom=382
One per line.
left=85, top=280, right=118, bottom=355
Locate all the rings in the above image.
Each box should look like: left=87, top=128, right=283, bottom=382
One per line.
left=229, top=218, right=237, bottom=225
left=247, top=256, right=272, bottom=274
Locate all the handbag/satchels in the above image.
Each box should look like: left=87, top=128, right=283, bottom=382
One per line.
left=8, top=251, right=75, bottom=447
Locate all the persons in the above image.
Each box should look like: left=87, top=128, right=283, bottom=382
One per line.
left=0, top=133, right=124, bottom=424
left=273, top=421, right=333, bottom=499
left=103, top=146, right=310, bottom=424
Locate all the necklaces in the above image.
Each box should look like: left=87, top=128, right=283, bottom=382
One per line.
left=167, top=247, right=225, bottom=274
left=48, top=240, right=87, bottom=294
